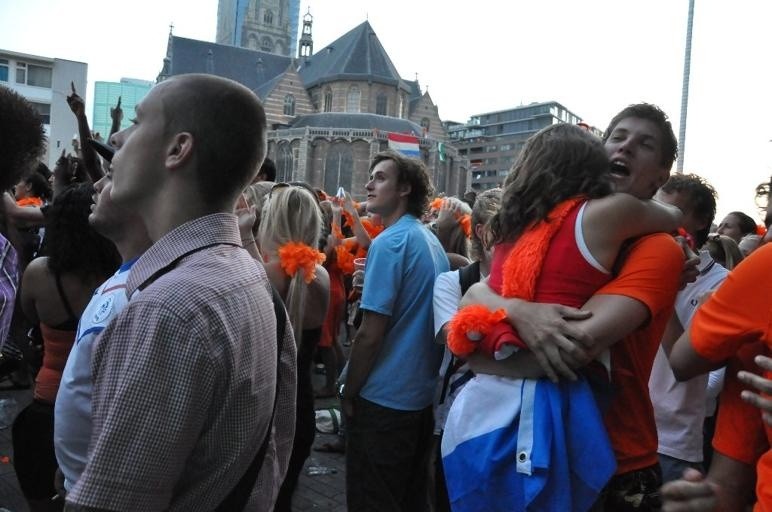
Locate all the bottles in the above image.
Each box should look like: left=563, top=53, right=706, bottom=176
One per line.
left=307, top=467, right=338, bottom=475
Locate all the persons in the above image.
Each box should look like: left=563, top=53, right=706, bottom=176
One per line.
left=0, top=73, right=771, bottom=511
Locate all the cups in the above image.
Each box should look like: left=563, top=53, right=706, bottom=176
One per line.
left=354, top=257, right=367, bottom=294
